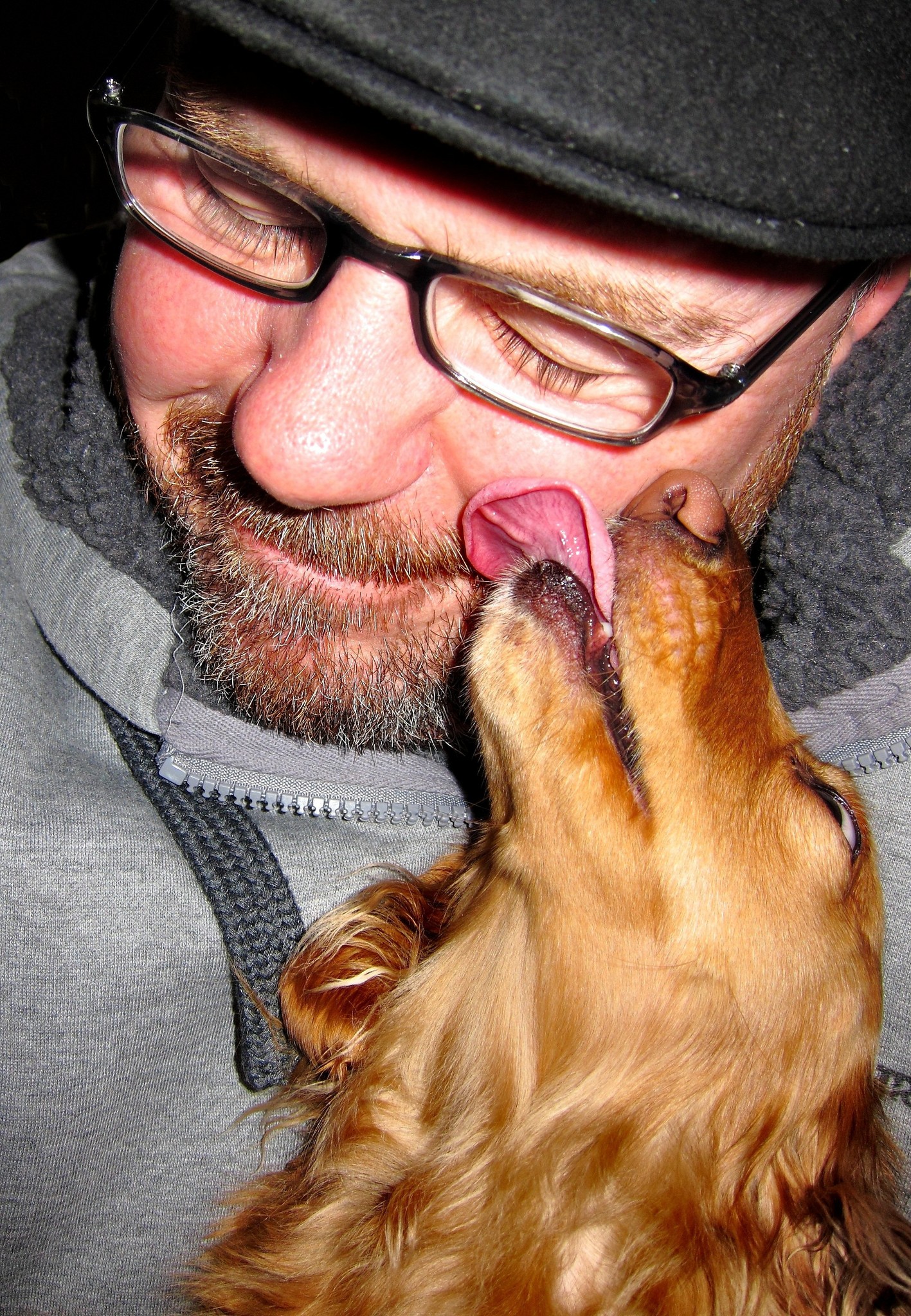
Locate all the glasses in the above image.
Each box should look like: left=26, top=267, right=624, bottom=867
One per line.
left=84, top=48, right=881, bottom=453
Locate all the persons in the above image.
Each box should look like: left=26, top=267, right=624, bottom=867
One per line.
left=0, top=2, right=911, bottom=1316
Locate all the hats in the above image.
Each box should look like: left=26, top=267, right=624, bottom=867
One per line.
left=177, top=1, right=911, bottom=262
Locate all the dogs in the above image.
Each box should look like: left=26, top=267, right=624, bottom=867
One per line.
left=180, top=470, right=911, bottom=1315
